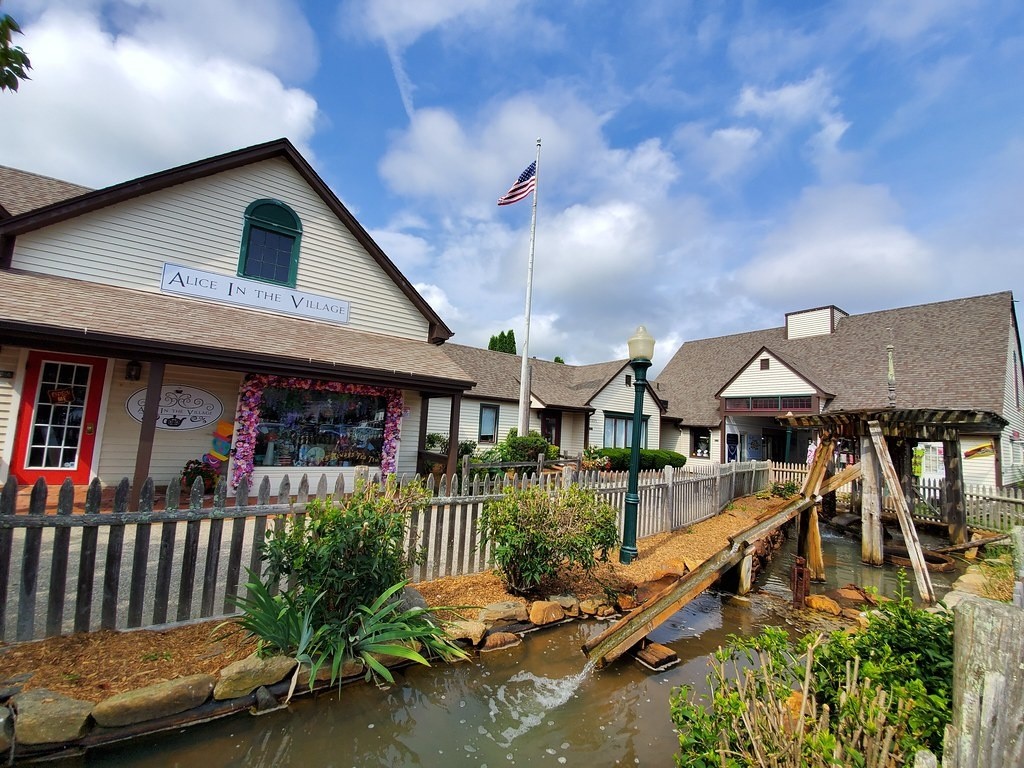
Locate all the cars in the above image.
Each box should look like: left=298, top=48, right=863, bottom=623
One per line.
left=257, top=422, right=383, bottom=454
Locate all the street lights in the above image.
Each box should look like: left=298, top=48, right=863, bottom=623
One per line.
left=620, top=325, right=656, bottom=565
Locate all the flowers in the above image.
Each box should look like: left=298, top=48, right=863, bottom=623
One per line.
left=179, top=460, right=218, bottom=490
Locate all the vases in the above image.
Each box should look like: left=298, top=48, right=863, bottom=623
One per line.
left=184, top=486, right=192, bottom=494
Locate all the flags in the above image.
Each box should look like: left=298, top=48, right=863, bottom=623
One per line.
left=498, top=160, right=537, bottom=205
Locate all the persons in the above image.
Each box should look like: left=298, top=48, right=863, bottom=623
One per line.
left=806, top=441, right=818, bottom=464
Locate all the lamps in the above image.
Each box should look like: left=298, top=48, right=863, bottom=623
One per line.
left=126, top=362, right=142, bottom=381
